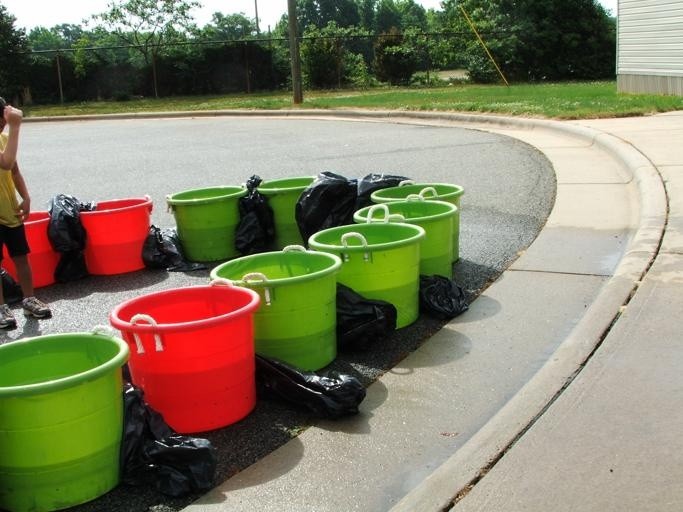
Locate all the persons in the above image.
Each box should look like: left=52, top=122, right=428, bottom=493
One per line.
left=0, top=97, right=52, bottom=328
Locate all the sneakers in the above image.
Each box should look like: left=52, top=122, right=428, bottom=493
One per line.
left=20, top=295, right=51, bottom=318
left=0, top=304, right=16, bottom=330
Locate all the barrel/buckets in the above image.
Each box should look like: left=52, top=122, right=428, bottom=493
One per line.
left=370, top=179, right=465, bottom=265
left=308, top=205, right=426, bottom=331
left=0, top=326, right=131, bottom=511
left=1, top=210, right=62, bottom=290
left=209, top=245, right=342, bottom=373
left=167, top=182, right=249, bottom=263
left=78, top=195, right=153, bottom=276
left=110, top=279, right=261, bottom=437
left=255, top=177, right=316, bottom=250
left=353, top=194, right=457, bottom=281
